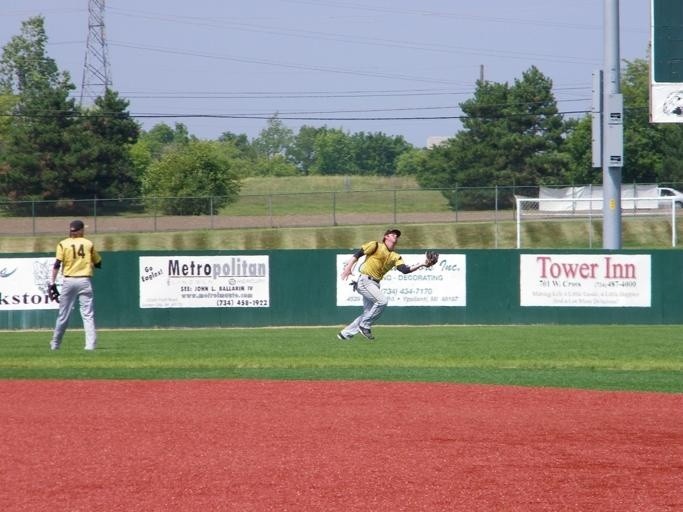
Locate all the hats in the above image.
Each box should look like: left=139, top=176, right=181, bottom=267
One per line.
left=69, top=220, right=89, bottom=232
left=385, top=229, right=401, bottom=237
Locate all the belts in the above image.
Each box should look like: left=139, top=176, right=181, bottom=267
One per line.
left=368, top=276, right=380, bottom=284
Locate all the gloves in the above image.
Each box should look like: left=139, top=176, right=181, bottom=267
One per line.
left=348, top=280, right=357, bottom=292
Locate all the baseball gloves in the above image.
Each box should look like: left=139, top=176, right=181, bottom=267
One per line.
left=46, top=284, right=62, bottom=303
left=424, top=249, right=439, bottom=269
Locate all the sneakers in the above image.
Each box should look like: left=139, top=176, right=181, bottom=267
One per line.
left=337, top=332, right=346, bottom=340
left=357, top=323, right=375, bottom=340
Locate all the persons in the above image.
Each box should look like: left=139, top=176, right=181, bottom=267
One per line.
left=336, top=227, right=438, bottom=342
left=47, top=219, right=103, bottom=350
